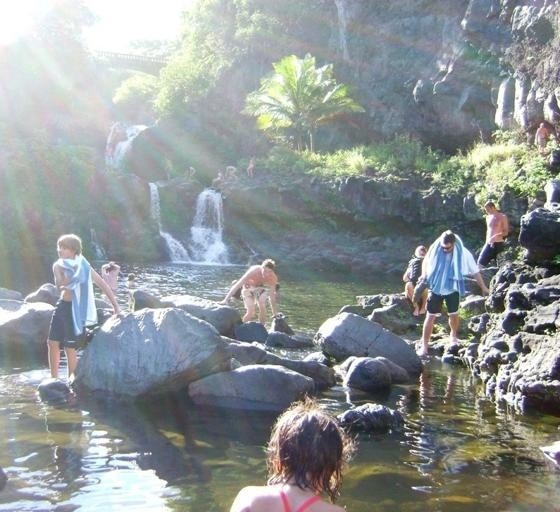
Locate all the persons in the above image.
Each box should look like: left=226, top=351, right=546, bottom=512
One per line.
left=476, top=201, right=509, bottom=268
left=220, top=258, right=277, bottom=325
left=402, top=244, right=427, bottom=316
left=46, top=232, right=120, bottom=377
left=227, top=396, right=348, bottom=512
left=523, top=121, right=534, bottom=145
left=100, top=261, right=121, bottom=303
left=535, top=123, right=549, bottom=152
left=411, top=227, right=488, bottom=356
left=229, top=278, right=282, bottom=300
left=127, top=273, right=139, bottom=310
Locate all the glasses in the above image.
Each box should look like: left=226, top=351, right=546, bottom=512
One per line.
left=441, top=245, right=452, bottom=250
